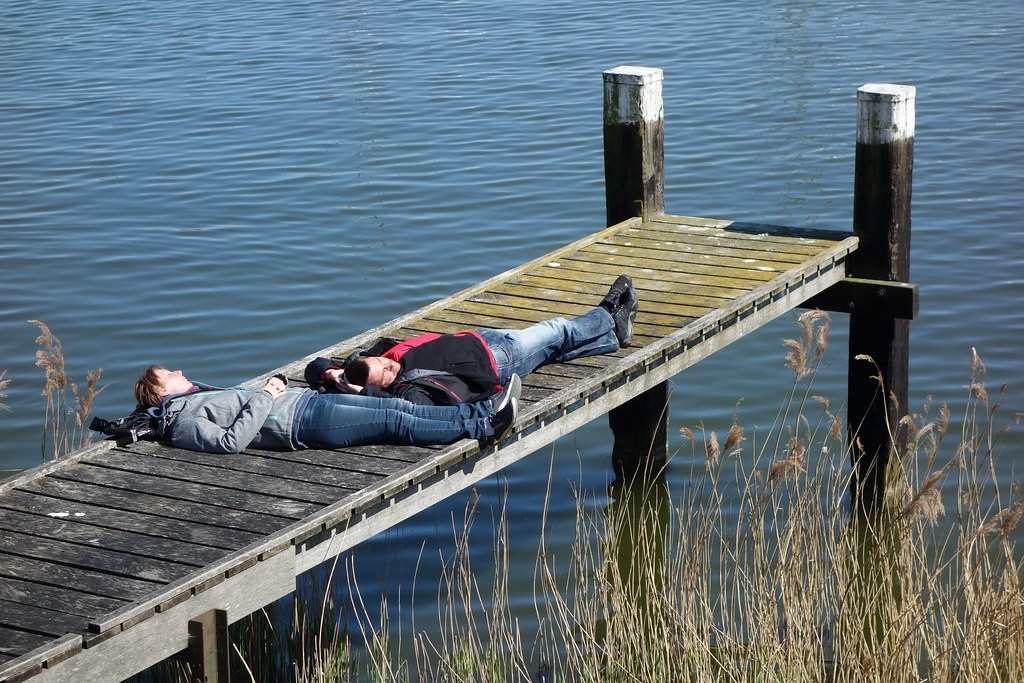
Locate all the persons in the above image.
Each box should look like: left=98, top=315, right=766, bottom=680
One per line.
left=305, top=274, right=638, bottom=407
left=134, top=365, right=521, bottom=454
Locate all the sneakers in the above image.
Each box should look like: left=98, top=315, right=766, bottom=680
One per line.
left=598, top=274, right=634, bottom=316
left=613, top=288, right=638, bottom=348
left=487, top=397, right=518, bottom=446
left=488, top=373, right=521, bottom=414
